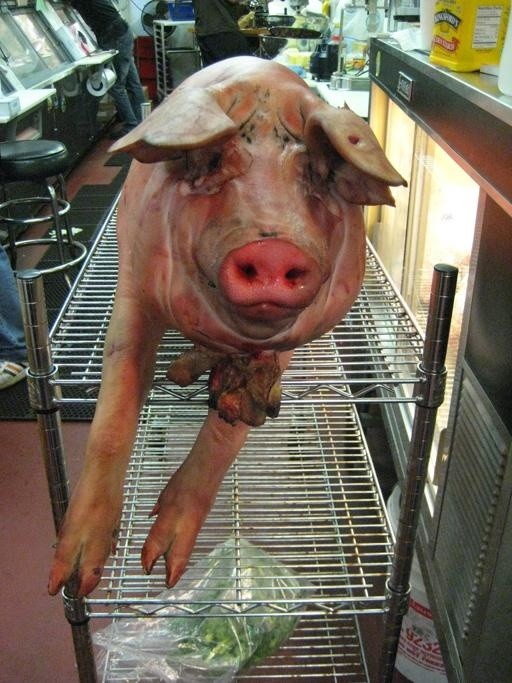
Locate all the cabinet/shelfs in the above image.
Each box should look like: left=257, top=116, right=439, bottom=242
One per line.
left=153, top=16, right=204, bottom=100
left=15, top=150, right=458, bottom=682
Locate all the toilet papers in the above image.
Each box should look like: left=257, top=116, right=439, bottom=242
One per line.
left=86, top=68, right=117, bottom=97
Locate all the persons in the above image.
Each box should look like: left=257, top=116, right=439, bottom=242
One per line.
left=0, top=245, right=29, bottom=387
left=193, top=0, right=250, bottom=68
left=57, top=0, right=144, bottom=137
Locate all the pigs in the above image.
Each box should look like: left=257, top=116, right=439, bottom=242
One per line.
left=45, top=53, right=410, bottom=597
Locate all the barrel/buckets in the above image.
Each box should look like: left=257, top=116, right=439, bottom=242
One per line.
left=382, top=481, right=449, bottom=683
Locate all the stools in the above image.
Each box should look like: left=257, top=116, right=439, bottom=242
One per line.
left=0, top=140, right=82, bottom=301
